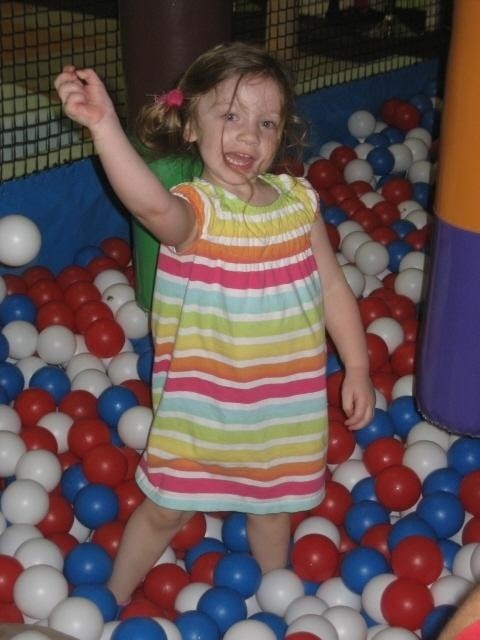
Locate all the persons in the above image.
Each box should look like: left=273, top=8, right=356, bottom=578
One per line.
left=55, top=40, right=376, bottom=619
